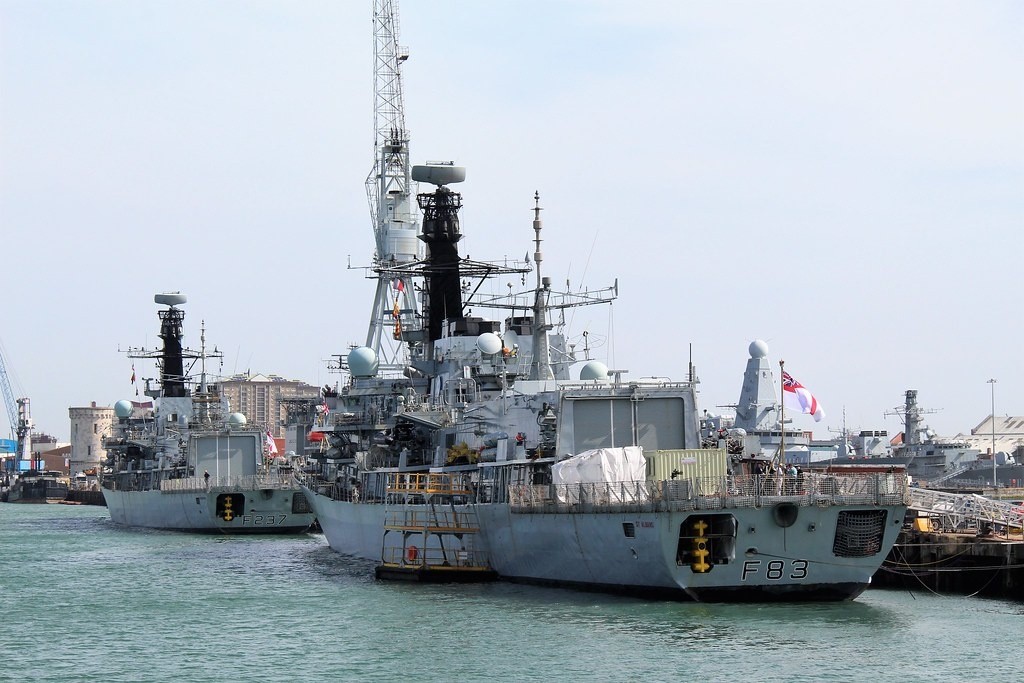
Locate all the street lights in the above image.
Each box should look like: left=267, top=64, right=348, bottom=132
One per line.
left=986, top=378, right=999, bottom=487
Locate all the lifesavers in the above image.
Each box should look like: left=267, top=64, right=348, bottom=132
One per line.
left=771, top=501, right=799, bottom=528
left=408, top=545, right=419, bottom=562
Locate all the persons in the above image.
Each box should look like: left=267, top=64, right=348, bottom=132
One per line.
left=774, top=463, right=804, bottom=496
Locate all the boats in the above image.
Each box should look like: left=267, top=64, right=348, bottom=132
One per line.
left=287, top=0, right=916, bottom=605
left=94, top=292, right=317, bottom=537
left=0, top=396, right=71, bottom=505
left=372, top=472, right=502, bottom=585
left=698, top=339, right=1024, bottom=498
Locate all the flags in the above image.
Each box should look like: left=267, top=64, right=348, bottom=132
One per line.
left=322, top=401, right=330, bottom=416
left=783, top=371, right=826, bottom=422
left=394, top=319, right=401, bottom=338
left=131, top=366, right=135, bottom=381
left=393, top=298, right=400, bottom=317
left=394, top=279, right=407, bottom=296
left=267, top=432, right=278, bottom=454
